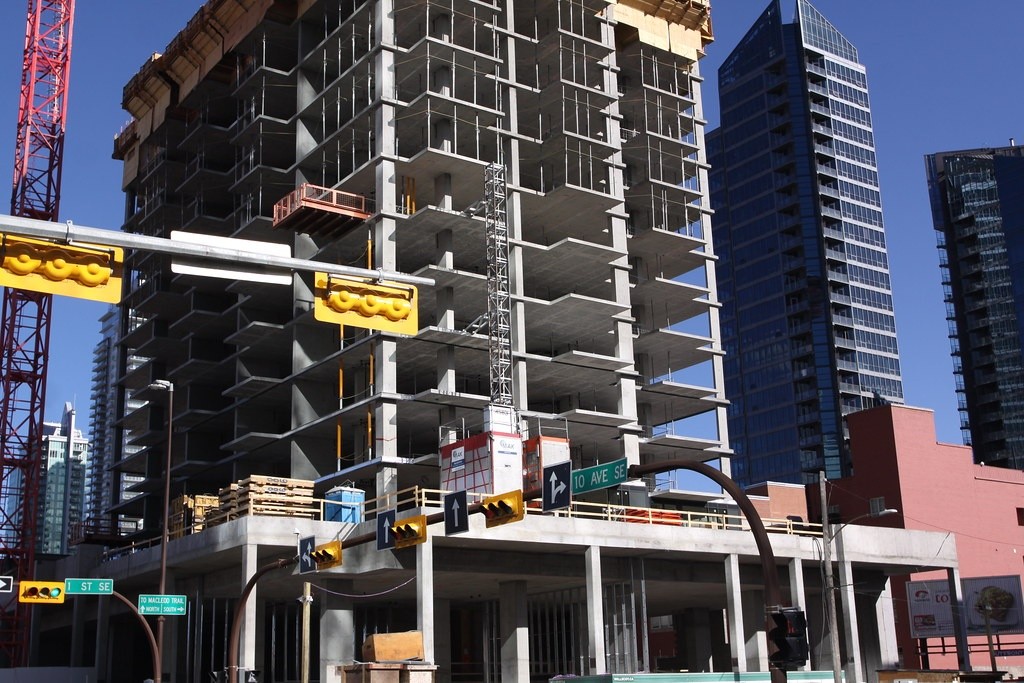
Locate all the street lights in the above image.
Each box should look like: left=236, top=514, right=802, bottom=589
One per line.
left=822, top=508, right=899, bottom=683
left=147, top=380, right=176, bottom=683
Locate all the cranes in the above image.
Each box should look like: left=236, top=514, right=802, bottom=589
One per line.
left=0, top=0, right=75, bottom=660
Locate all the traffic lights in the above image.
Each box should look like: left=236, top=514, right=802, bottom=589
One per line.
left=18, top=581, right=65, bottom=603
left=479, top=489, right=523, bottom=528
left=388, top=515, right=427, bottom=550
left=310, top=540, right=342, bottom=572
left=770, top=611, right=810, bottom=661
